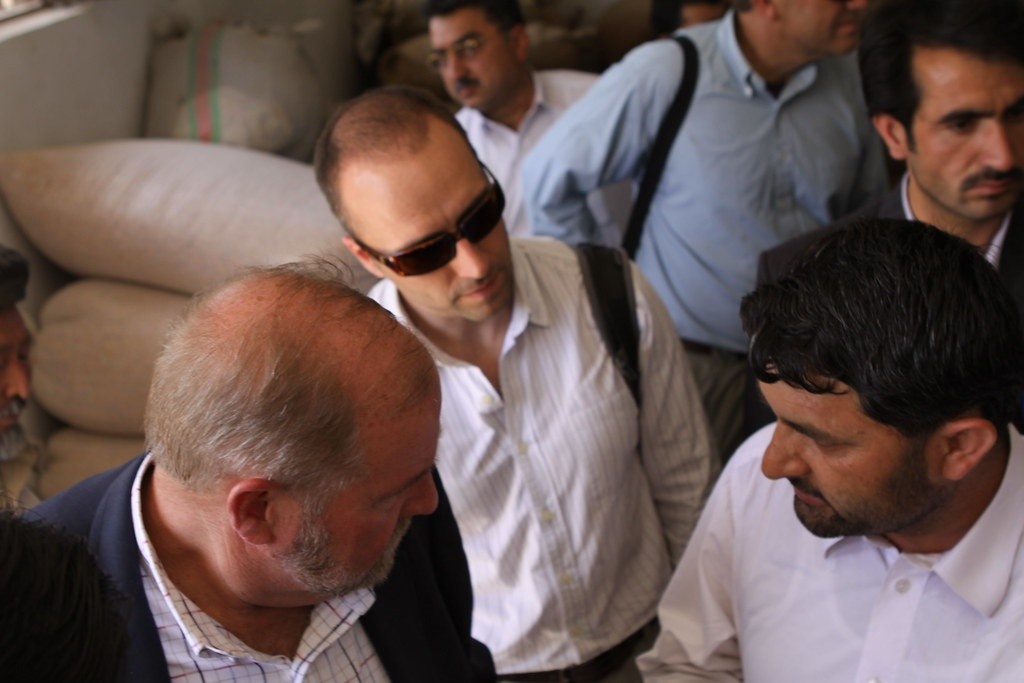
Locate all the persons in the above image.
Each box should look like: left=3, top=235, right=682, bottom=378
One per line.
left=312, top=85, right=721, bottom=683
left=0, top=246, right=500, bottom=683
left=427, top=0, right=1024, bottom=464
left=632, top=219, right=1024, bottom=682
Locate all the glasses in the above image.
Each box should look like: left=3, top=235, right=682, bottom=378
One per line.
left=351, top=160, right=506, bottom=276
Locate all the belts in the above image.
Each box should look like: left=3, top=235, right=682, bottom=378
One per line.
left=680, top=337, right=748, bottom=366
left=496, top=617, right=658, bottom=683
